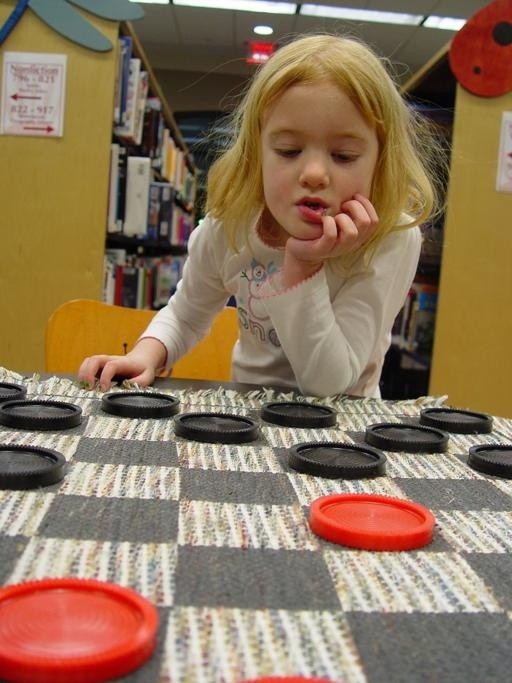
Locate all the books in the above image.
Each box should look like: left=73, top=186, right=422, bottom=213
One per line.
left=391, top=226, right=445, bottom=372
left=104, top=35, right=197, bottom=311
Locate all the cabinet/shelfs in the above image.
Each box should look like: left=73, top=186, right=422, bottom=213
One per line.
left=377, top=30, right=512, bottom=421
left=0, top=0, right=198, bottom=379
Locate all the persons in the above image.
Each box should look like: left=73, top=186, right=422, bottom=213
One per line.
left=79, top=33, right=452, bottom=395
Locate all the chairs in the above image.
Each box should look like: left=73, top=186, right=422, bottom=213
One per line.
left=44, top=297, right=242, bottom=383
